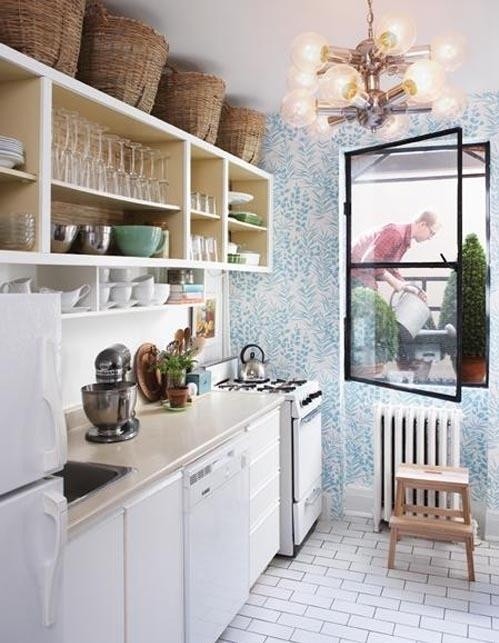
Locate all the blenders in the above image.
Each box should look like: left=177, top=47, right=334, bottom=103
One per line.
left=80, top=343, right=139, bottom=443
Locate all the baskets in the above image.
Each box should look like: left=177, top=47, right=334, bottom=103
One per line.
left=154, top=63, right=228, bottom=147
left=88, top=1, right=170, bottom=115
left=219, top=104, right=266, bottom=165
left=0, top=0, right=85, bottom=81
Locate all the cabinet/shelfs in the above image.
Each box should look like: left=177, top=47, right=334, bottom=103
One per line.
left=67, top=472, right=185, bottom=643
left=283, top=0, right=466, bottom=140
left=185, top=411, right=280, bottom=643
left=0, top=45, right=274, bottom=317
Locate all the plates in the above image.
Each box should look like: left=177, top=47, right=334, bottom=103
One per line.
left=62, top=305, right=92, bottom=310
left=1, top=135, right=24, bottom=169
left=1, top=214, right=37, bottom=250
left=100, top=301, right=117, bottom=309
left=101, top=283, right=117, bottom=288
left=118, top=299, right=138, bottom=307
left=114, top=281, right=138, bottom=287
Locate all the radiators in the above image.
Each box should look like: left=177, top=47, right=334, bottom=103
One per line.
left=371, top=407, right=461, bottom=534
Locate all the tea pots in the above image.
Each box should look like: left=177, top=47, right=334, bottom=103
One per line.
left=238, top=344, right=270, bottom=381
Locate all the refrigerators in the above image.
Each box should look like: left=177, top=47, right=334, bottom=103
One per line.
left=1, top=294, right=69, bottom=641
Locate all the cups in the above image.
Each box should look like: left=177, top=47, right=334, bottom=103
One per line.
left=191, top=191, right=200, bottom=210
left=99, top=266, right=110, bottom=282
left=52, top=103, right=170, bottom=204
left=190, top=233, right=218, bottom=261
left=98, top=287, right=110, bottom=303
left=199, top=191, right=207, bottom=211
left=39, top=282, right=91, bottom=309
left=205, top=193, right=216, bottom=213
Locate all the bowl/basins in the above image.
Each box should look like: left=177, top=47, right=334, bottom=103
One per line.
left=111, top=224, right=169, bottom=257
left=110, top=286, right=132, bottom=302
left=168, top=269, right=186, bottom=284
left=79, top=223, right=112, bottom=254
left=110, top=269, right=130, bottom=282
left=50, top=221, right=80, bottom=252
left=154, top=282, right=169, bottom=304
left=130, top=273, right=154, bottom=305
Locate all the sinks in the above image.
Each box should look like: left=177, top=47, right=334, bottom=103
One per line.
left=52, top=461, right=133, bottom=508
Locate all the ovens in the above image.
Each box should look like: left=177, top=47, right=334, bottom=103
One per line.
left=291, top=404, right=323, bottom=545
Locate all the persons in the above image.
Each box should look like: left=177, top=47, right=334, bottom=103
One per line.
left=346, top=210, right=440, bottom=297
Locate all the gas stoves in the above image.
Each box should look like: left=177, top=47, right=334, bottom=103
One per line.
left=211, top=376, right=323, bottom=418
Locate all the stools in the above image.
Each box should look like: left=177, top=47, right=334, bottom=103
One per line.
left=393, top=466, right=478, bottom=582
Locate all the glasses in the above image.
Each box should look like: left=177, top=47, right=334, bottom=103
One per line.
left=428, top=227, right=438, bottom=237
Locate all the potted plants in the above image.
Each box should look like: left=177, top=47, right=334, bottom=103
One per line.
left=351, top=289, right=398, bottom=377
left=441, top=234, right=490, bottom=384
